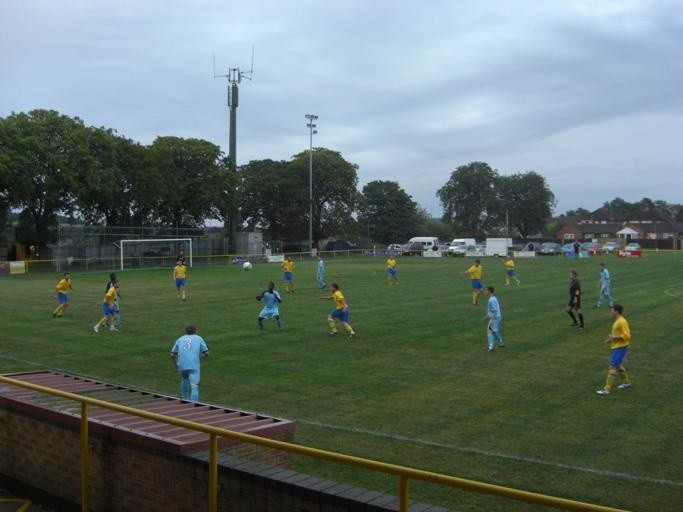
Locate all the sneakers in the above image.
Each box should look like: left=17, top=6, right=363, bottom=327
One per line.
left=92, top=324, right=101, bottom=332
left=109, top=326, right=119, bottom=332
left=497, top=342, right=504, bottom=347
left=349, top=331, right=357, bottom=338
left=330, top=328, right=336, bottom=336
left=598, top=389, right=611, bottom=394
left=488, top=346, right=496, bottom=352
left=618, top=382, right=632, bottom=389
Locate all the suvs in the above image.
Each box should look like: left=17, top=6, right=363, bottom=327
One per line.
left=323, top=240, right=357, bottom=257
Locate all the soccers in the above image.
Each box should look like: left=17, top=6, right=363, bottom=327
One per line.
left=243, top=262, right=252, bottom=271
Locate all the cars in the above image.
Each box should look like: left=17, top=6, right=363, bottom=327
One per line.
left=386, top=237, right=621, bottom=256
left=625, top=243, right=640, bottom=251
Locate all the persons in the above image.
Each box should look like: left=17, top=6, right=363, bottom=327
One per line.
left=501, top=253, right=522, bottom=286
left=168, top=321, right=209, bottom=401
left=482, top=284, right=503, bottom=353
left=93, top=279, right=119, bottom=333
left=170, top=255, right=401, bottom=332
left=593, top=262, right=615, bottom=309
left=50, top=271, right=74, bottom=319
left=320, top=281, right=355, bottom=338
left=565, top=270, right=584, bottom=328
left=464, top=259, right=484, bottom=307
left=595, top=303, right=632, bottom=394
left=104, top=273, right=121, bottom=328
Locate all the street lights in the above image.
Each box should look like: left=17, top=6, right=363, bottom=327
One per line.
left=305, top=114, right=319, bottom=257
